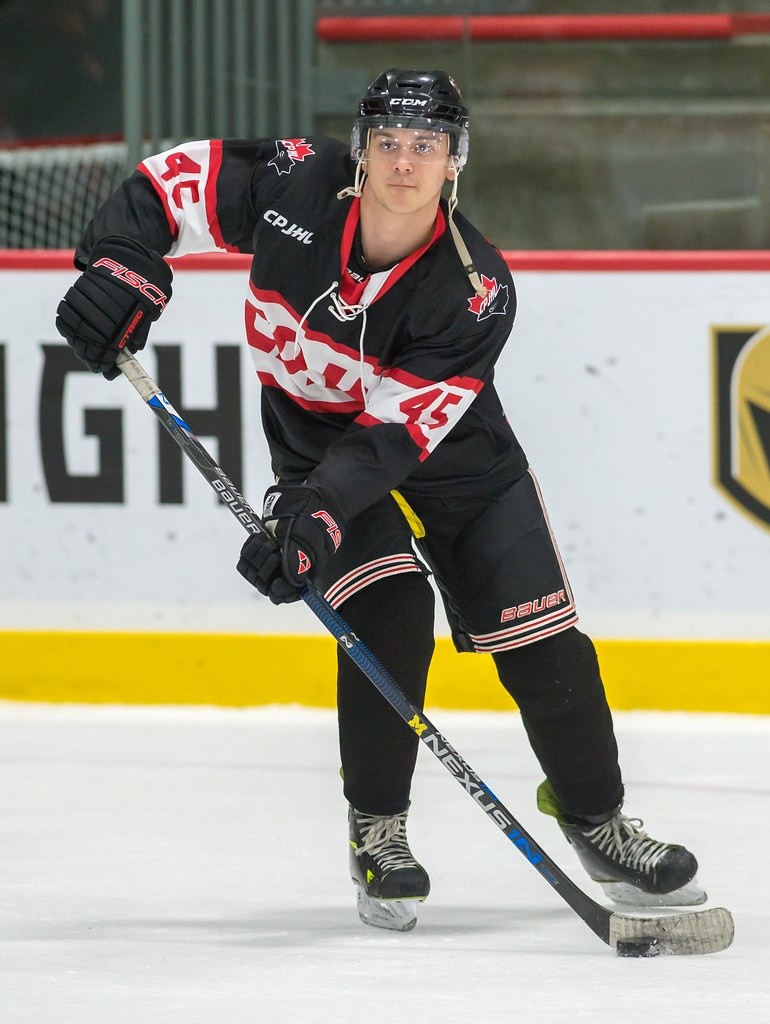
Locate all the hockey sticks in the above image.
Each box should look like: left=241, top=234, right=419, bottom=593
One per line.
left=117, top=352, right=737, bottom=960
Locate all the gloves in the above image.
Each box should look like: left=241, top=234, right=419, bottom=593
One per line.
left=236, top=483, right=350, bottom=602
left=55, top=257, right=172, bottom=380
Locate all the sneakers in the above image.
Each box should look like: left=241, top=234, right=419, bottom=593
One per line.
left=537, top=779, right=706, bottom=906
left=349, top=801, right=429, bottom=933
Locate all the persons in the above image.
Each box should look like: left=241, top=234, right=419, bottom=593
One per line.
left=56, top=69, right=708, bottom=929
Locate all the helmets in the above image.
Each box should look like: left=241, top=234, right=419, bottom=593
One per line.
left=355, top=70, right=464, bottom=154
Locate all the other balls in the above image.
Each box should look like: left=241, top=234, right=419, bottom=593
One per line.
left=617, top=935, right=661, bottom=957
